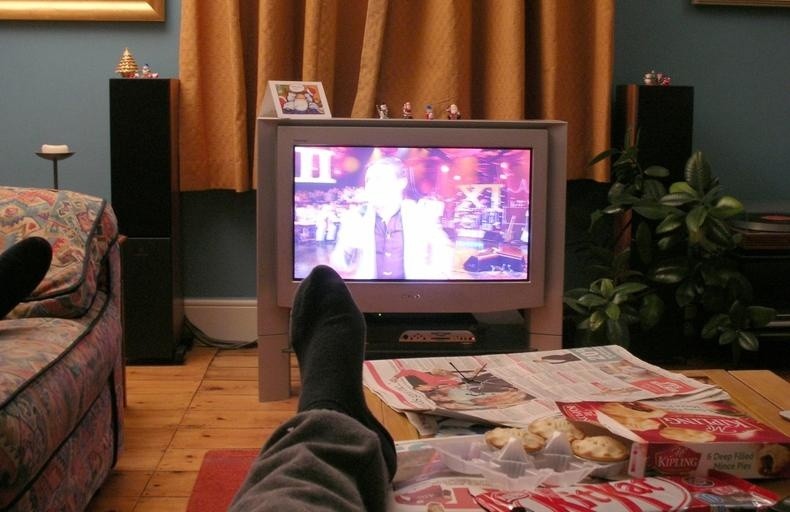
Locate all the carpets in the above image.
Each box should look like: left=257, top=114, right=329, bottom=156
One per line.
left=186, top=447, right=262, bottom=512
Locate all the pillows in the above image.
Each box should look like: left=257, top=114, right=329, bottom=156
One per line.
left=1, top=183, right=120, bottom=320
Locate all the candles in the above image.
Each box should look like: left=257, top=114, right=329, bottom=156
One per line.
left=41, top=143, right=69, bottom=153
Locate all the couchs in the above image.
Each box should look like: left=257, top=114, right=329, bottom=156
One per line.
left=0, top=234, right=127, bottom=512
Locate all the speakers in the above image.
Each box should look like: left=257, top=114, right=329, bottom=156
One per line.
left=108, top=78, right=186, bottom=365
left=616, top=83, right=695, bottom=364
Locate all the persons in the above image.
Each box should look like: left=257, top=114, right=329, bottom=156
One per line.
left=218, top=264, right=398, bottom=511
left=424, top=104, right=434, bottom=120
left=298, top=185, right=445, bottom=247
left=374, top=102, right=390, bottom=118
left=445, top=103, right=462, bottom=120
left=321, top=157, right=459, bottom=282
left=385, top=366, right=534, bottom=412
left=399, top=101, right=414, bottom=118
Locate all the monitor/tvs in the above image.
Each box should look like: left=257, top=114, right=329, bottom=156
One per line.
left=275, top=124, right=549, bottom=316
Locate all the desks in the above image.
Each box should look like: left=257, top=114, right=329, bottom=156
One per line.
left=359, top=367, right=789, bottom=512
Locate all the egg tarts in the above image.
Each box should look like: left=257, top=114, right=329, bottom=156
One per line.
left=756, top=444, right=789, bottom=472
left=660, top=426, right=717, bottom=444
left=571, top=436, right=628, bottom=463
left=528, top=412, right=585, bottom=439
left=487, top=422, right=543, bottom=453
left=598, top=398, right=665, bottom=433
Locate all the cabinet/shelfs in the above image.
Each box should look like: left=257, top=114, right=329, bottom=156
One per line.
left=711, top=248, right=790, bottom=370
